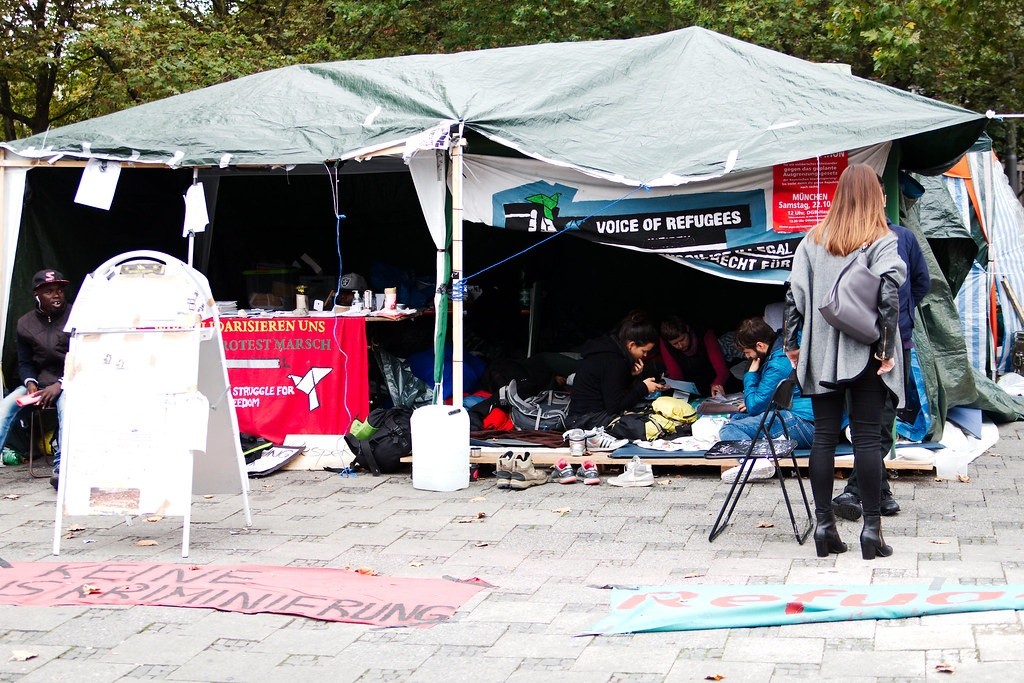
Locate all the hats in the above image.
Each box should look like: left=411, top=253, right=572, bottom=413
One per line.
left=33, top=269, right=68, bottom=289
left=333, top=272, right=367, bottom=293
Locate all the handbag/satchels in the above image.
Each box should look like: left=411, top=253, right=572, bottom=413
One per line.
left=818, top=234, right=881, bottom=344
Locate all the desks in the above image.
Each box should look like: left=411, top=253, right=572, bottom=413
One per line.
left=220, top=309, right=418, bottom=470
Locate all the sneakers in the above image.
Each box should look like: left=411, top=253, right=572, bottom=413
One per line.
left=550, top=458, right=576, bottom=484
left=510, top=454, right=550, bottom=489
left=879, top=489, right=898, bottom=513
left=575, top=458, right=600, bottom=484
left=607, top=454, right=654, bottom=486
left=562, top=428, right=585, bottom=456
left=584, top=427, right=629, bottom=451
left=721, top=457, right=777, bottom=484
left=832, top=492, right=862, bottom=520
left=492, top=452, right=513, bottom=488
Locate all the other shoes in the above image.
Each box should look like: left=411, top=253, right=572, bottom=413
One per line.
left=50, top=474, right=58, bottom=489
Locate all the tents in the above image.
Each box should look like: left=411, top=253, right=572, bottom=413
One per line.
left=0, top=25, right=1024, bottom=408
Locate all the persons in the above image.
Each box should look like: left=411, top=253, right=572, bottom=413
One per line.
left=659, top=316, right=733, bottom=400
left=565, top=316, right=668, bottom=441
left=830, top=176, right=931, bottom=521
left=720, top=319, right=814, bottom=483
left=339, top=272, right=394, bottom=405
left=791, top=164, right=907, bottom=559
left=0, top=269, right=73, bottom=489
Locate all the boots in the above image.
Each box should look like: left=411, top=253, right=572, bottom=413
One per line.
left=815, top=510, right=848, bottom=557
left=861, top=514, right=893, bottom=561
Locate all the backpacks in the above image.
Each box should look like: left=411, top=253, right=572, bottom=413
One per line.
left=344, top=405, right=415, bottom=476
left=498, top=379, right=571, bottom=431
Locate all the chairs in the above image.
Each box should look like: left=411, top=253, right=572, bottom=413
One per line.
left=26, top=405, right=57, bottom=478
left=703, top=378, right=813, bottom=545
